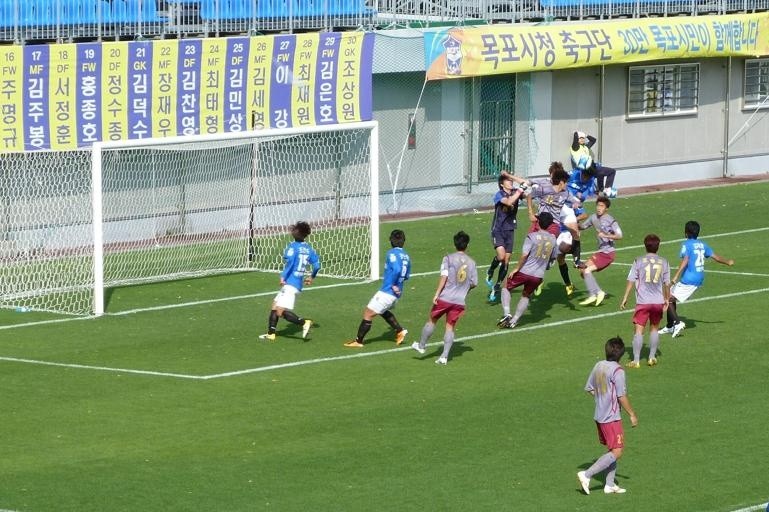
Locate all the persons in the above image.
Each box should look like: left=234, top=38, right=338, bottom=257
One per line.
left=577, top=196, right=623, bottom=307
left=657, top=221, right=734, bottom=338
left=570, top=132, right=617, bottom=198
left=342, top=229, right=411, bottom=348
left=577, top=338, right=638, bottom=495
left=497, top=212, right=557, bottom=328
left=410, top=231, right=478, bottom=365
left=620, top=234, right=671, bottom=368
left=500, top=161, right=586, bottom=270
left=573, top=189, right=585, bottom=202
left=258, top=221, right=321, bottom=340
left=555, top=189, right=589, bottom=296
left=567, top=168, right=597, bottom=199
left=526, top=170, right=581, bottom=297
left=485, top=175, right=539, bottom=304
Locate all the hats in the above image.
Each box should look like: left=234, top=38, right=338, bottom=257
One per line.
left=578, top=131, right=585, bottom=138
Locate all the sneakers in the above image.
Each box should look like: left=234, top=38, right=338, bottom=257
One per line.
left=534, top=281, right=544, bottom=296
left=603, top=186, right=613, bottom=196
left=595, top=290, right=607, bottom=306
left=625, top=360, right=640, bottom=369
left=565, top=282, right=574, bottom=296
left=572, top=260, right=587, bottom=268
left=672, top=320, right=686, bottom=339
left=302, top=319, right=312, bottom=338
left=648, top=357, right=657, bottom=366
left=434, top=356, right=448, bottom=365
left=577, top=471, right=591, bottom=495
left=411, top=340, right=425, bottom=354
left=496, top=313, right=513, bottom=328
left=598, top=192, right=606, bottom=198
left=396, top=329, right=408, bottom=347
left=657, top=325, right=674, bottom=334
left=488, top=292, right=496, bottom=303
left=258, top=333, right=276, bottom=340
left=343, top=341, right=364, bottom=347
left=485, top=268, right=493, bottom=287
left=504, top=321, right=515, bottom=328
left=604, top=485, right=627, bottom=494
left=547, top=260, right=555, bottom=270
left=579, top=295, right=597, bottom=305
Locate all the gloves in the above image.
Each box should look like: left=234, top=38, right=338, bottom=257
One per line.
left=519, top=182, right=528, bottom=191
